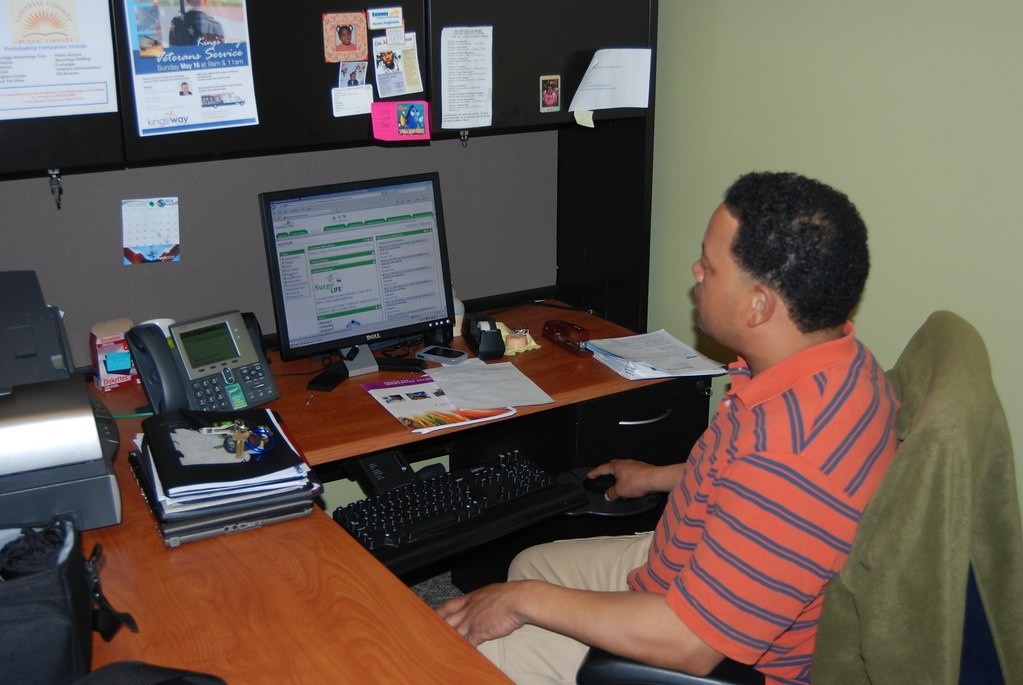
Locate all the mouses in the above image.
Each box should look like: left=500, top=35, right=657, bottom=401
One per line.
left=584, top=473, right=616, bottom=494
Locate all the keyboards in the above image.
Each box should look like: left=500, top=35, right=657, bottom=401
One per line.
left=333, top=448, right=583, bottom=564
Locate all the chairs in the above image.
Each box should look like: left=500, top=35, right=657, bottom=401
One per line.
left=572, top=311, right=990, bottom=685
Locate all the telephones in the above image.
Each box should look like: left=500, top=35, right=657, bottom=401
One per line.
left=124, top=309, right=281, bottom=415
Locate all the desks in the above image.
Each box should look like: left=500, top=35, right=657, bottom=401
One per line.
left=81, top=374, right=522, bottom=685
left=263, top=297, right=684, bottom=470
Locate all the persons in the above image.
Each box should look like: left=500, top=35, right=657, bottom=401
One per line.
left=336, top=26, right=356, bottom=51
left=544, top=84, right=558, bottom=107
left=179, top=82, right=193, bottom=96
left=168, top=0, right=226, bottom=45
left=435, top=172, right=903, bottom=685
left=347, top=72, right=358, bottom=86
left=377, top=51, right=400, bottom=74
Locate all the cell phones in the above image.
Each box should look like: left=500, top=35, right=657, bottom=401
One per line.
left=415, top=345, right=468, bottom=365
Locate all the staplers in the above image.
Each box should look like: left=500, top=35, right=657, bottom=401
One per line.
left=542, top=319, right=594, bottom=357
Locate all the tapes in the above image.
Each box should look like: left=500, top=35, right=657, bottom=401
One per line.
left=476, top=320, right=490, bottom=330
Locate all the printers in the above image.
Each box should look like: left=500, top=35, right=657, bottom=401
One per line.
left=0, top=269, right=125, bottom=549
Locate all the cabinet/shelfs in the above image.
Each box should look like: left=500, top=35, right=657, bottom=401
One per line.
left=110, top=0, right=433, bottom=169
left=426, top=1, right=659, bottom=140
left=569, top=375, right=713, bottom=541
left=0, top=0, right=126, bottom=182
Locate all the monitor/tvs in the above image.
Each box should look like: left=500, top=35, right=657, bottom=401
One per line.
left=258, top=173, right=456, bottom=362
